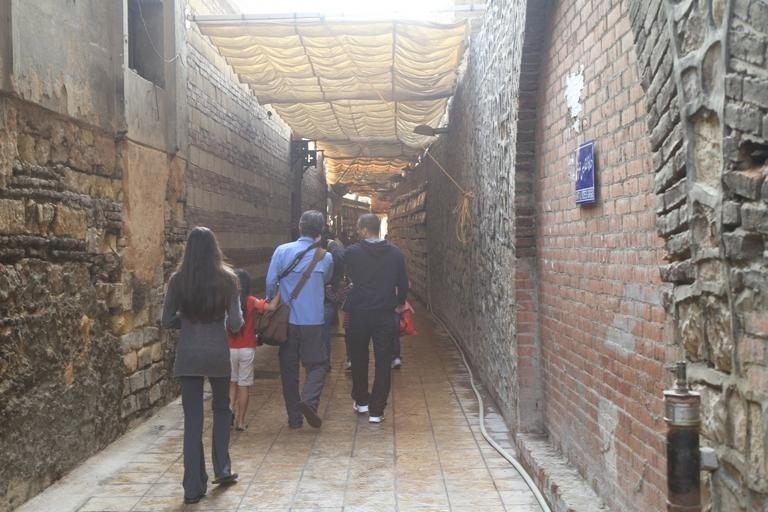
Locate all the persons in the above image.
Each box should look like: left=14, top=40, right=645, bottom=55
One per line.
left=326, top=214, right=408, bottom=424
left=162, top=227, right=246, bottom=504
left=320, top=232, right=402, bottom=372
left=223, top=267, right=280, bottom=430
left=265, top=211, right=336, bottom=427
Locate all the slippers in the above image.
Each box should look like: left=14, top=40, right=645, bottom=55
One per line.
left=183, top=485, right=208, bottom=504
left=210, top=473, right=238, bottom=484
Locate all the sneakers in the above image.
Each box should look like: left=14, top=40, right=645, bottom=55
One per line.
left=296, top=400, right=321, bottom=428
left=390, top=358, right=402, bottom=370
left=343, top=362, right=352, bottom=373
left=352, top=399, right=369, bottom=413
left=368, top=414, right=384, bottom=423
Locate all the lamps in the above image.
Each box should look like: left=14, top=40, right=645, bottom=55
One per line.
left=412, top=123, right=451, bottom=138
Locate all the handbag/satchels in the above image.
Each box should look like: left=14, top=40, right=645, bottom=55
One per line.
left=254, top=305, right=290, bottom=346
